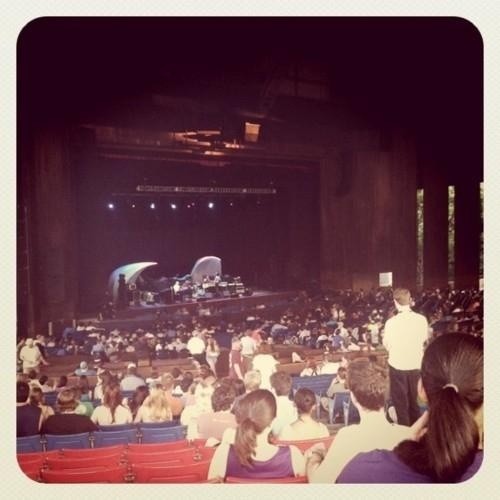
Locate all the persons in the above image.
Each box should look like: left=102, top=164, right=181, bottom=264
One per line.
left=382, top=287, right=431, bottom=425
left=304, top=357, right=412, bottom=483
left=335, top=331, right=484, bottom=483
left=410, top=288, right=484, bottom=336
left=15, top=273, right=393, bottom=440
left=207, top=389, right=303, bottom=479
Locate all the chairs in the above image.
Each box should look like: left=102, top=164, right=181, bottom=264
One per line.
left=17, top=289, right=484, bottom=485
